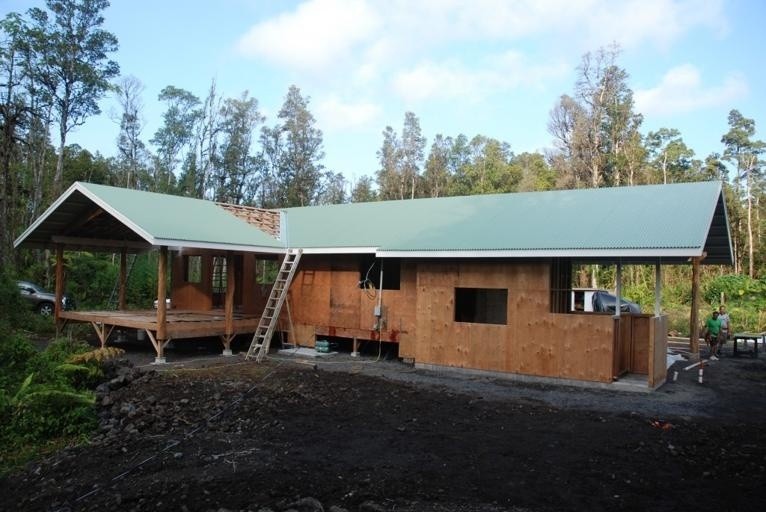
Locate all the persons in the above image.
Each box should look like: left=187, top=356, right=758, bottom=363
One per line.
left=717, top=305, right=732, bottom=356
left=699, top=312, right=721, bottom=358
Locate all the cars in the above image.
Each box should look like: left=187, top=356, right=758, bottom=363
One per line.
left=16, top=280, right=76, bottom=318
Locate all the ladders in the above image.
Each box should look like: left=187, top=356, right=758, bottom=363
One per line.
left=245, top=247, right=303, bottom=363
left=106, top=254, right=137, bottom=310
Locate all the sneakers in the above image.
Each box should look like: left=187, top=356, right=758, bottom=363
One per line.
left=710, top=350, right=722, bottom=362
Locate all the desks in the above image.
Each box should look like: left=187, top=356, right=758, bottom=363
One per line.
left=734, top=333, right=766, bottom=359
